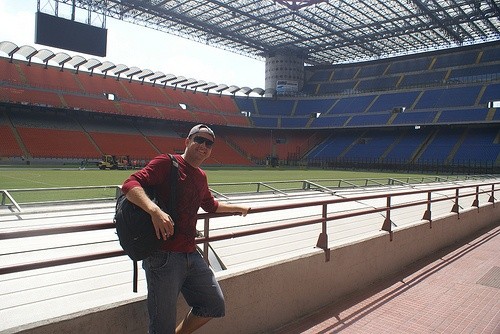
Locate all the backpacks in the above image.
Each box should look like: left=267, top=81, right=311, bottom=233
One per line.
left=113, top=153, right=178, bottom=293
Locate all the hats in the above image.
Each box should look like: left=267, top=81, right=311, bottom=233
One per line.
left=188, top=124, right=217, bottom=140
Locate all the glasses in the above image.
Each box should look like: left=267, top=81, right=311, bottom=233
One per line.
left=194, top=136, right=214, bottom=148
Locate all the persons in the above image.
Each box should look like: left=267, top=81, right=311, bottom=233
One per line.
left=122, top=157, right=149, bottom=170
left=121, top=123, right=252, bottom=334
left=21, top=154, right=25, bottom=161
